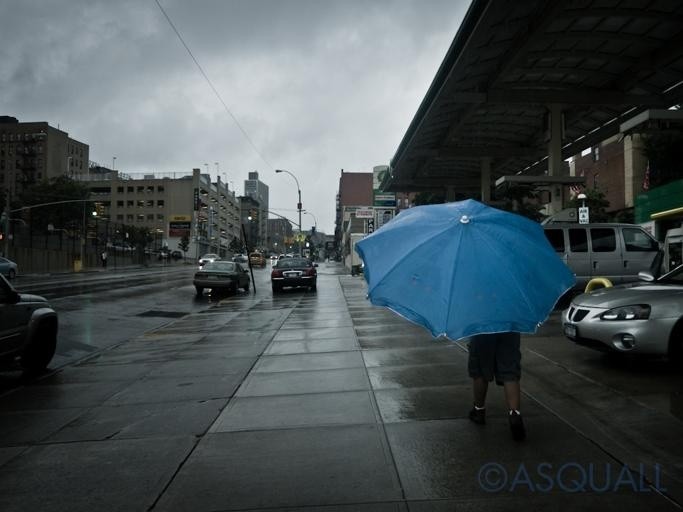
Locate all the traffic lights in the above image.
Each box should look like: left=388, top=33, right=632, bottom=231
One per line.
left=99, top=203, right=105, bottom=215
left=248, top=210, right=252, bottom=222
left=253, top=211, right=257, bottom=222
left=94, top=204, right=98, bottom=215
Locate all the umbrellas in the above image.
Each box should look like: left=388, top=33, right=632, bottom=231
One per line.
left=354, top=198, right=578, bottom=342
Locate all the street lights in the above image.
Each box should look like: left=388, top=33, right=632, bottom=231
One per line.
left=68, top=157, right=73, bottom=175
left=275, top=169, right=303, bottom=258
left=261, top=209, right=299, bottom=229
left=112, top=157, right=118, bottom=171
left=203, top=161, right=235, bottom=192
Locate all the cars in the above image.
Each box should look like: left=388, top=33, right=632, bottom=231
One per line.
left=270, top=249, right=308, bottom=259
left=0, top=274, right=61, bottom=377
left=232, top=254, right=248, bottom=262
left=199, top=253, right=222, bottom=264
left=270, top=257, right=320, bottom=294
left=111, top=241, right=136, bottom=252
left=146, top=249, right=169, bottom=259
left=192, top=260, right=251, bottom=296
left=562, top=263, right=683, bottom=373
left=0, top=256, right=20, bottom=278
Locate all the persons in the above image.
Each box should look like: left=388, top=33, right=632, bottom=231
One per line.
left=467, top=332, right=526, bottom=442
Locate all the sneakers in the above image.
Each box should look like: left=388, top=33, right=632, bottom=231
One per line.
left=468, top=407, right=487, bottom=425
left=509, top=409, right=523, bottom=426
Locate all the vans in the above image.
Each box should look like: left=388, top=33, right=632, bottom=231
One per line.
left=248, top=253, right=266, bottom=267
left=536, top=223, right=665, bottom=310
